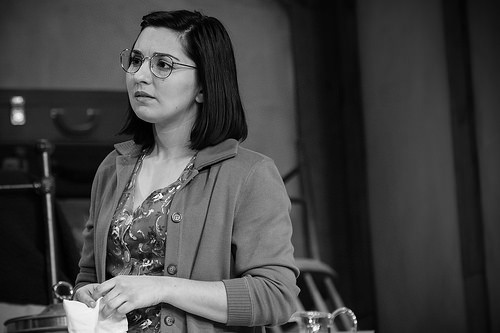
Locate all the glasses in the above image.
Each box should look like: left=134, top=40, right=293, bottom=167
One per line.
left=120, top=48, right=196, bottom=78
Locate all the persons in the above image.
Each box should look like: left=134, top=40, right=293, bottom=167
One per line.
left=69, top=9, right=300, bottom=333
